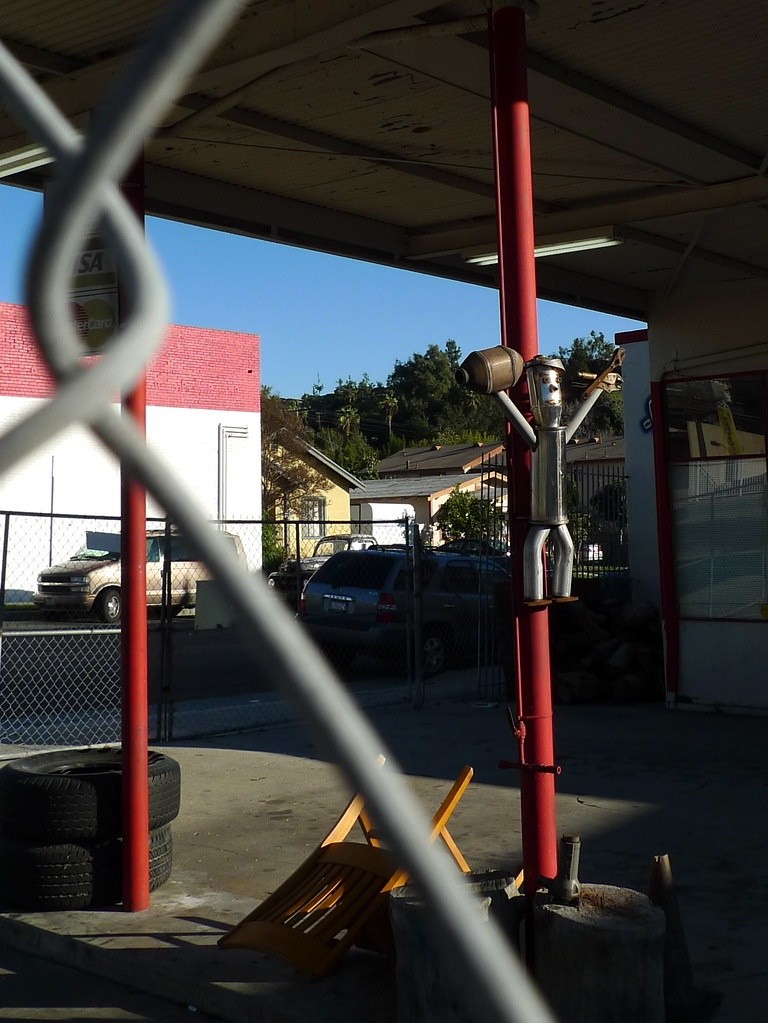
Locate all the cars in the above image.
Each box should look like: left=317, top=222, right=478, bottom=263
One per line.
left=439, top=540, right=554, bottom=582
left=268, top=534, right=380, bottom=596
left=577, top=543, right=603, bottom=561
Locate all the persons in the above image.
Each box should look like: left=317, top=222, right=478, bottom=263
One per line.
left=456, top=347, right=610, bottom=599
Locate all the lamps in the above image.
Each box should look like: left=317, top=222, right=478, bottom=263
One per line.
left=461, top=225, right=626, bottom=268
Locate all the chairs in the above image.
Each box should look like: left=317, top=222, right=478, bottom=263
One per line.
left=217, top=763, right=473, bottom=980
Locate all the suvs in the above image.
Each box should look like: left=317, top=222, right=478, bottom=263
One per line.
left=291, top=545, right=510, bottom=668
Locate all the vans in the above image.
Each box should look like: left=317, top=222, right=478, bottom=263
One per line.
left=31, top=529, right=236, bottom=623
